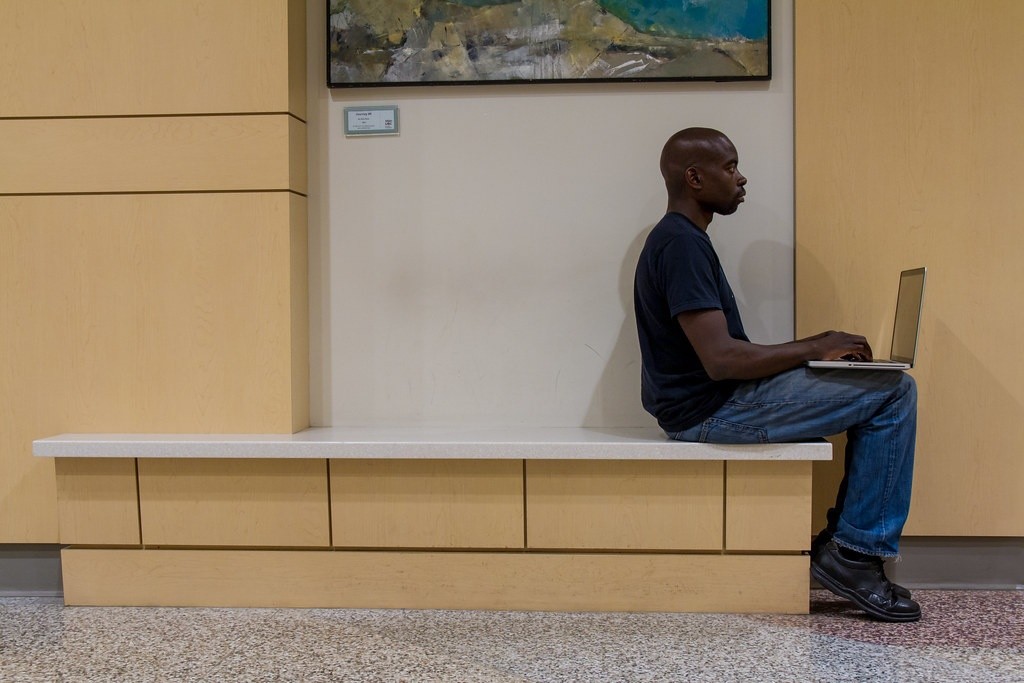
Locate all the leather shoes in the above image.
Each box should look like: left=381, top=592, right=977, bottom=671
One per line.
left=812, top=531, right=912, bottom=600
left=813, top=541, right=923, bottom=621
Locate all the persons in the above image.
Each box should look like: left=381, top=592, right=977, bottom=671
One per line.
left=631, top=124, right=926, bottom=626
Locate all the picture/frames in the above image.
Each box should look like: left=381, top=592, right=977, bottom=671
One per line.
left=323, top=0, right=773, bottom=88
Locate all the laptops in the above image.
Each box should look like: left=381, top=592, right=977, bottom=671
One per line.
left=807, top=267, right=928, bottom=369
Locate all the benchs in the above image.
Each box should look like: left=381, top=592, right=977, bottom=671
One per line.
left=31, top=425, right=835, bottom=613
